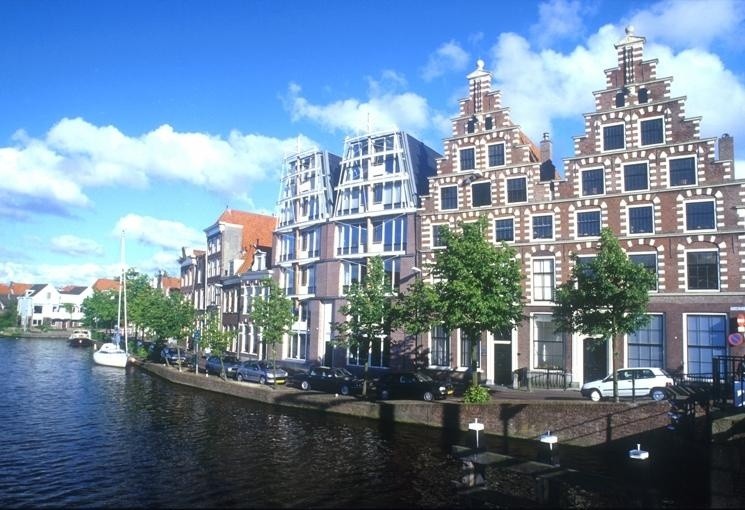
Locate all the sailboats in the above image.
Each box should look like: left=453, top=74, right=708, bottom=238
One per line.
left=93, top=226, right=136, bottom=367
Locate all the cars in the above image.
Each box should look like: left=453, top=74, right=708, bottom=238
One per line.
left=97, top=331, right=189, bottom=364
left=234, top=360, right=287, bottom=388
left=289, top=365, right=376, bottom=397
left=577, top=367, right=677, bottom=402
left=369, top=369, right=454, bottom=401
left=204, top=353, right=242, bottom=380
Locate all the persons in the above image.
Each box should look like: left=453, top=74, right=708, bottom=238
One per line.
left=737, top=354, right=745, bottom=381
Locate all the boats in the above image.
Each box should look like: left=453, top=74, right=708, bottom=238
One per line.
left=68, top=329, right=94, bottom=347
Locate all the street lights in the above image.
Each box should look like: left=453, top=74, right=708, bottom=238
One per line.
left=410, top=260, right=424, bottom=370
